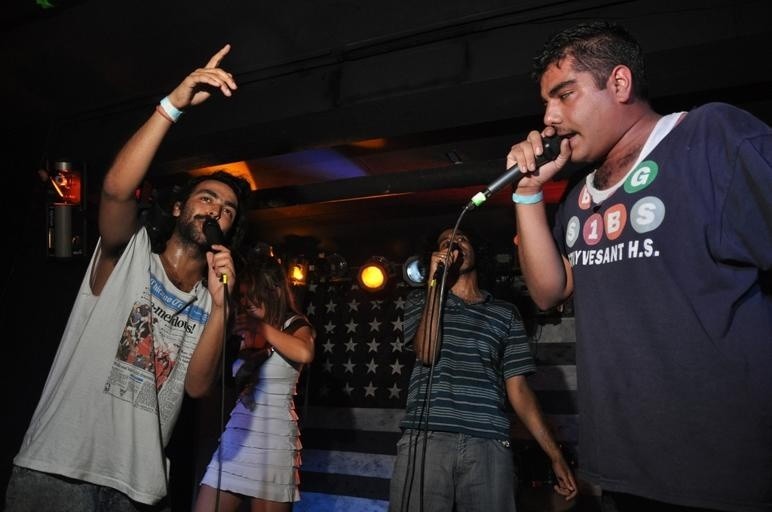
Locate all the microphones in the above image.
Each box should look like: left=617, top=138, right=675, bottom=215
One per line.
left=470, top=135, right=562, bottom=210
left=433, top=240, right=460, bottom=280
left=202, top=214, right=226, bottom=275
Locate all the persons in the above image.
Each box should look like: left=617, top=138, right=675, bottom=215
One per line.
left=193, top=254, right=319, bottom=512
left=3, top=40, right=236, bottom=512
left=506, top=21, right=770, bottom=512
left=386, top=225, right=577, bottom=511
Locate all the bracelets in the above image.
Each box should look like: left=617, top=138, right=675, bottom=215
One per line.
left=156, top=105, right=174, bottom=124
left=160, top=96, right=183, bottom=122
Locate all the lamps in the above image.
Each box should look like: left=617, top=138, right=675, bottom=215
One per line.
left=306, top=252, right=428, bottom=295
